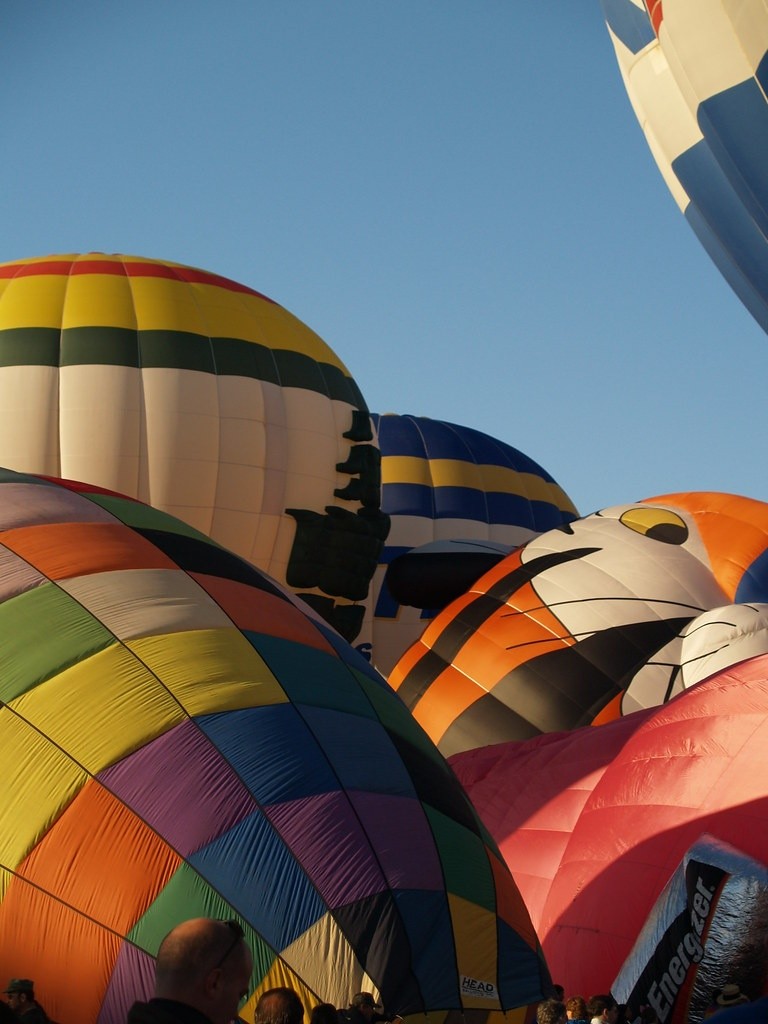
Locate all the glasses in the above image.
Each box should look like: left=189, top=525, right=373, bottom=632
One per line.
left=216, top=919, right=245, bottom=968
left=612, top=1008, right=618, bottom=1014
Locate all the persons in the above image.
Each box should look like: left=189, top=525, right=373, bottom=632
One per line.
left=0, top=979, right=48, bottom=1024
left=310, top=992, right=408, bottom=1024
left=702, top=997, right=768, bottom=1024
left=704, top=985, right=751, bottom=1019
left=254, top=987, right=304, bottom=1024
left=533, top=985, right=657, bottom=1024
left=127, top=918, right=252, bottom=1024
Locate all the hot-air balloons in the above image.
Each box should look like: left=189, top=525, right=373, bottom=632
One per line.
left=387, top=493, right=768, bottom=760
left=600, top=0, right=768, bottom=334
left=447, top=654, right=768, bottom=1024
left=351, top=413, right=580, bottom=681
left=0, top=251, right=391, bottom=644
left=1, top=466, right=558, bottom=1024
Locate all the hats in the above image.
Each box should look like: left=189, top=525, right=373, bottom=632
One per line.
left=716, top=984, right=747, bottom=1005
left=2, top=978, right=33, bottom=993
left=352, top=992, right=382, bottom=1008
